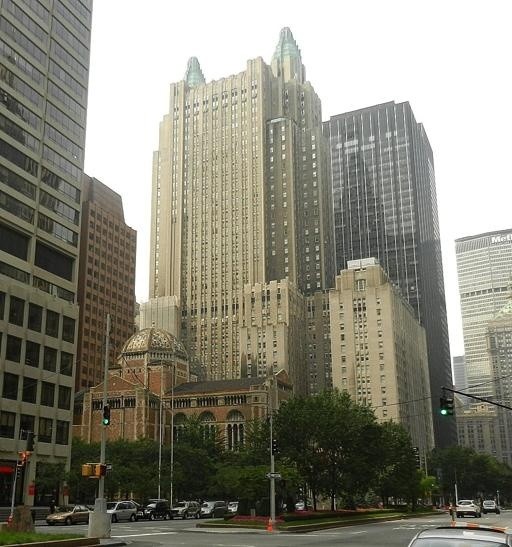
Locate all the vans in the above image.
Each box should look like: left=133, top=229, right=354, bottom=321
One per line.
left=201, top=500, right=228, bottom=518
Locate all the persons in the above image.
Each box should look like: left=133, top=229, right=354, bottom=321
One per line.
left=30, top=509, right=36, bottom=524
left=50, top=499, right=55, bottom=514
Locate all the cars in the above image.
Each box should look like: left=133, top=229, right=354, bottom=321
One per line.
left=228, top=501, right=239, bottom=513
left=106, top=500, right=138, bottom=521
left=408, top=520, right=511, bottom=546
left=295, top=500, right=313, bottom=510
left=46, top=504, right=94, bottom=525
left=122, top=500, right=142, bottom=507
left=86, top=504, right=95, bottom=510
left=450, top=505, right=456, bottom=515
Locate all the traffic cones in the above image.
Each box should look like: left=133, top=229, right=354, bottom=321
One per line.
left=267, top=519, right=273, bottom=531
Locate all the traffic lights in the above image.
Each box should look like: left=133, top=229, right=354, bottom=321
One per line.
left=447, top=398, right=453, bottom=415
left=82, top=464, right=95, bottom=476
left=22, top=451, right=28, bottom=465
left=95, top=464, right=106, bottom=476
left=440, top=396, right=446, bottom=415
left=104, top=404, right=111, bottom=425
left=26, top=430, right=36, bottom=451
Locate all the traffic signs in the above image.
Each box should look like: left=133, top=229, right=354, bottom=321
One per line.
left=265, top=471, right=280, bottom=478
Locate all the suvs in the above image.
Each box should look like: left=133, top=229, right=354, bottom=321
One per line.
left=455, top=499, right=481, bottom=517
left=137, top=498, right=172, bottom=520
left=170, top=500, right=202, bottom=518
left=483, top=499, right=498, bottom=514
